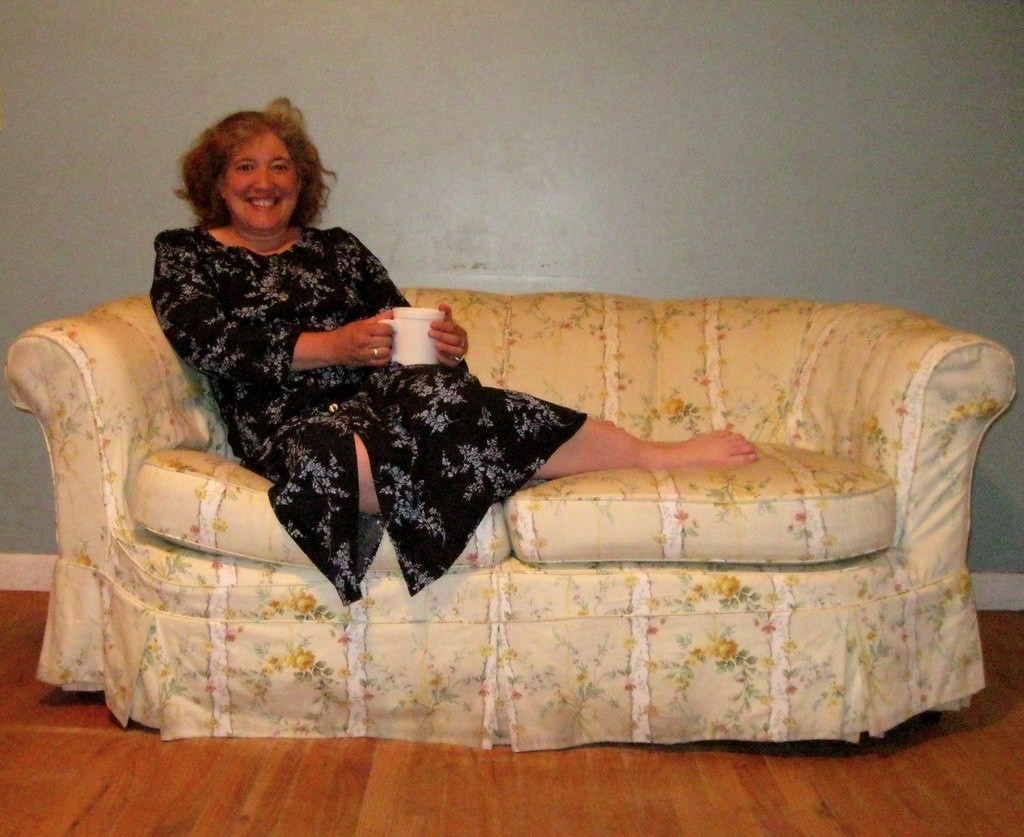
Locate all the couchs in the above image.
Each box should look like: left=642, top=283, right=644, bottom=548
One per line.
left=4, top=288, right=1016, bottom=750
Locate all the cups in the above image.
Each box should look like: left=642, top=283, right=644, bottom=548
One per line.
left=376, top=307, right=445, bottom=366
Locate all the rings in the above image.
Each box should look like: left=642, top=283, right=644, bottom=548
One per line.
left=454, top=355, right=465, bottom=362
left=373, top=348, right=378, bottom=357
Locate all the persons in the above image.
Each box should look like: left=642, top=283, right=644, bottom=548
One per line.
left=148, top=96, right=760, bottom=608
